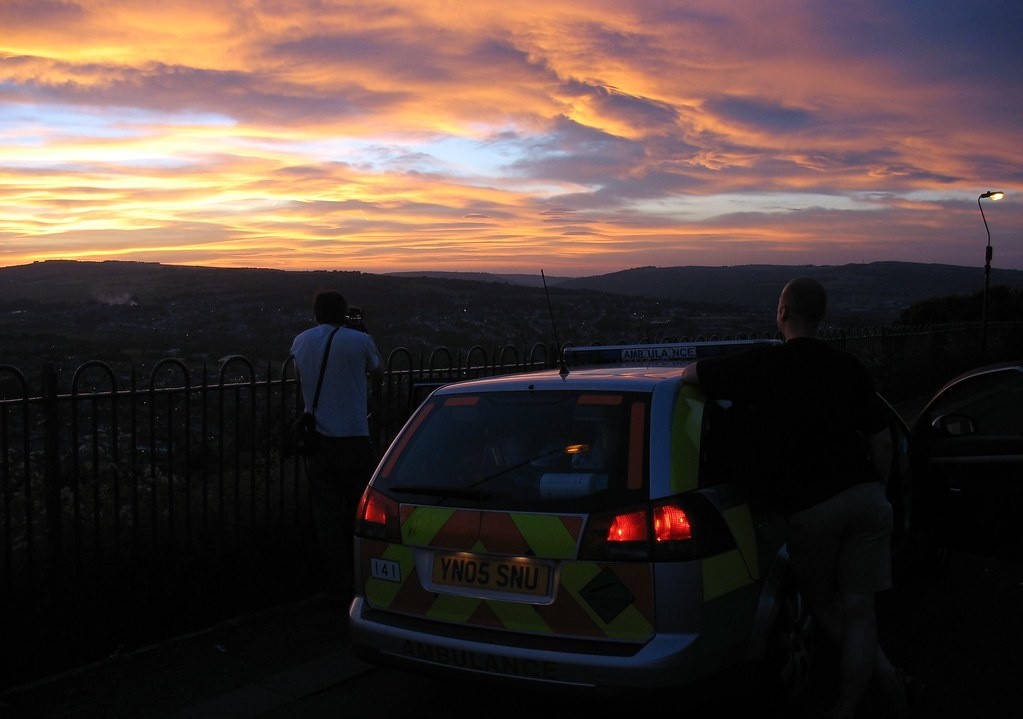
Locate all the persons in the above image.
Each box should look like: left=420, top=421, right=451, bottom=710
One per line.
left=289, top=292, right=385, bottom=602
left=683, top=276, right=895, bottom=719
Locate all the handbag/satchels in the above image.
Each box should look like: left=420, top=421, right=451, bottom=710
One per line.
left=288, top=412, right=320, bottom=457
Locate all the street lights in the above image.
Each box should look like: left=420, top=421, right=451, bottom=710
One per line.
left=974, top=189, right=1006, bottom=363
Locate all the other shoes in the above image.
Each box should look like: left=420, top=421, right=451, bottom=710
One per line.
left=888, top=669, right=928, bottom=719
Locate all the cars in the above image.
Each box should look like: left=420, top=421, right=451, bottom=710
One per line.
left=348, top=339, right=1023, bottom=719
